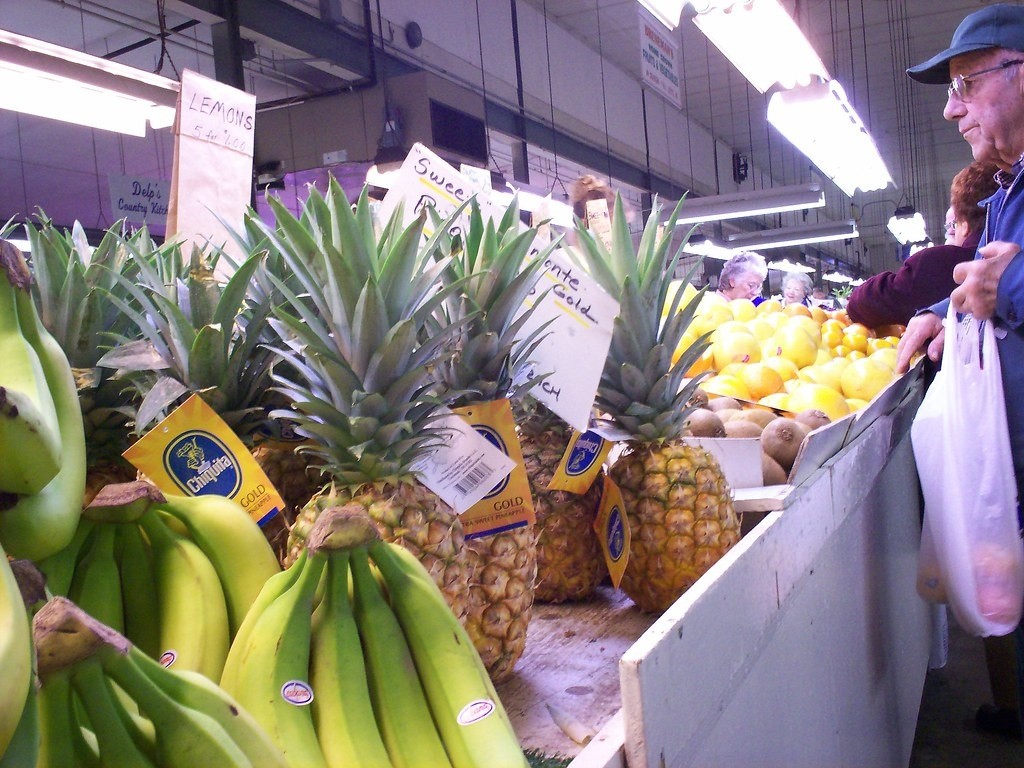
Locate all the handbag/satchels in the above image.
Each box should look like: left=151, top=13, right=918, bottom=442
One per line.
left=910, top=293, right=1024, bottom=637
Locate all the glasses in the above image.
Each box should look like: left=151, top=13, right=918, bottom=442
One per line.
left=945, top=59, right=1023, bottom=100
left=749, top=284, right=763, bottom=293
left=951, top=219, right=957, bottom=230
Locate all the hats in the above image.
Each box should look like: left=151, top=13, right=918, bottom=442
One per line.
left=906, top=4, right=1024, bottom=85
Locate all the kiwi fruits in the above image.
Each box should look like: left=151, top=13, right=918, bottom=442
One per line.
left=678, top=384, right=833, bottom=487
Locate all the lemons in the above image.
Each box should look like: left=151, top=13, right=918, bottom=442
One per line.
left=658, top=278, right=929, bottom=422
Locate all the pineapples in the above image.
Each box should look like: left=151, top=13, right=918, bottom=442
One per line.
left=0, top=184, right=743, bottom=688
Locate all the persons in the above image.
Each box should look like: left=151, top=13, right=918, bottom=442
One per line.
left=898, top=4, right=1024, bottom=740
left=550, top=176, right=634, bottom=252
left=717, top=159, right=1000, bottom=333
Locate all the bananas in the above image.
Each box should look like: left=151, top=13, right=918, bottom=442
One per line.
left=0, top=240, right=532, bottom=768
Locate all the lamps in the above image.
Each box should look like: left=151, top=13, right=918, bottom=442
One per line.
left=636, top=0, right=934, bottom=286
left=363, top=0, right=576, bottom=230
left=0, top=0, right=182, bottom=138
